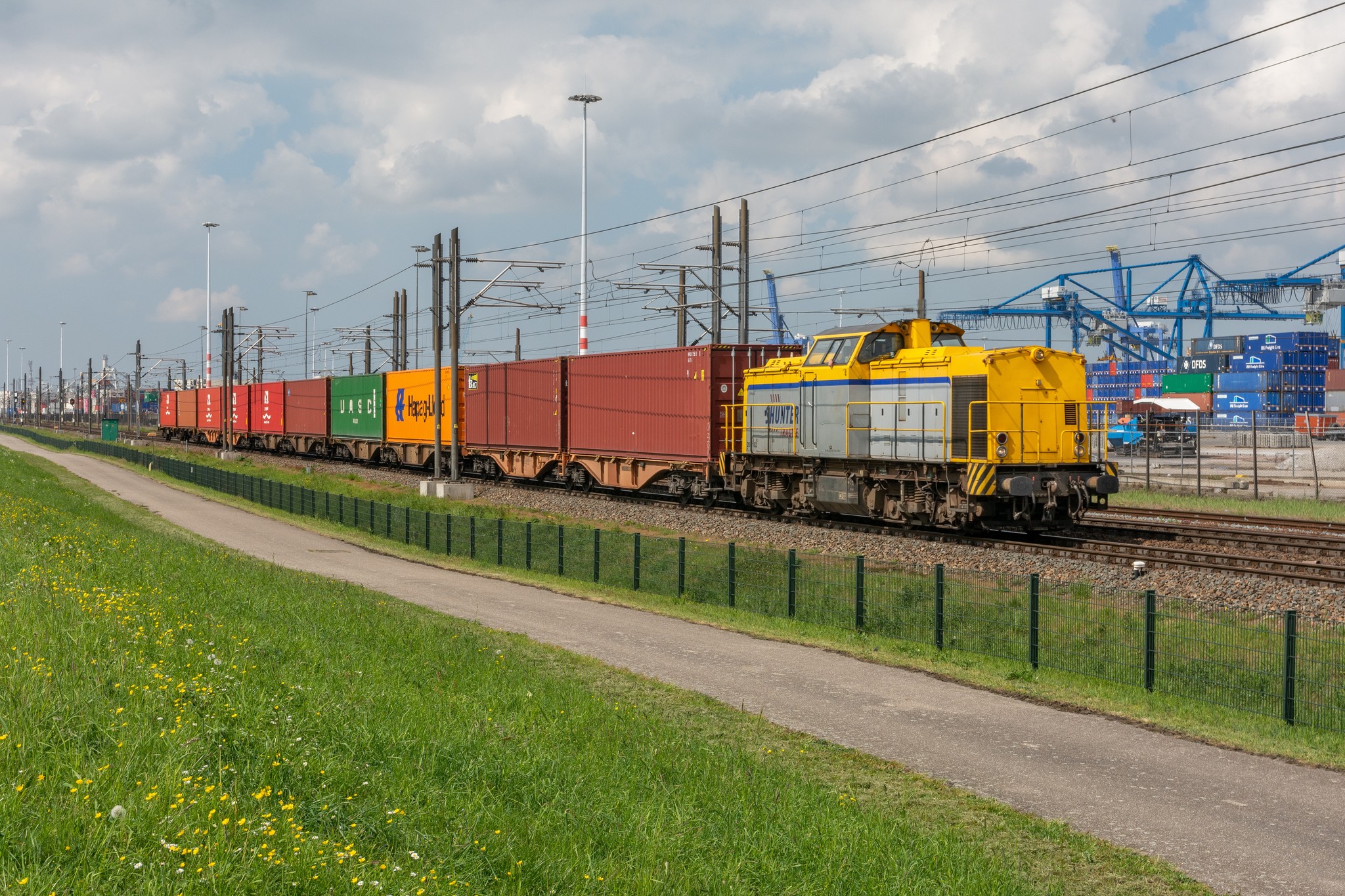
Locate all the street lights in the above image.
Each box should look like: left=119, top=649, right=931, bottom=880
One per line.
left=236, top=306, right=254, bottom=385
left=302, top=290, right=320, bottom=380
left=49, top=376, right=52, bottom=414
left=58, top=322, right=67, bottom=372
left=5, top=339, right=13, bottom=402
left=837, top=289, right=846, bottom=327
left=73, top=368, right=77, bottom=399
left=19, top=347, right=26, bottom=396
left=568, top=93, right=604, bottom=355
left=198, top=221, right=220, bottom=388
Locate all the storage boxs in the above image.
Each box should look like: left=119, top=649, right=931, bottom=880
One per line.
left=1085, top=332, right=1345, bottom=430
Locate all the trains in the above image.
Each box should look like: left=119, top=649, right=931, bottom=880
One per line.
left=158, top=270, right=1122, bottom=541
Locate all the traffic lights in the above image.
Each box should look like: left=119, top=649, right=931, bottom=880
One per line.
left=70, top=399, right=74, bottom=405
left=21, top=398, right=25, bottom=404
